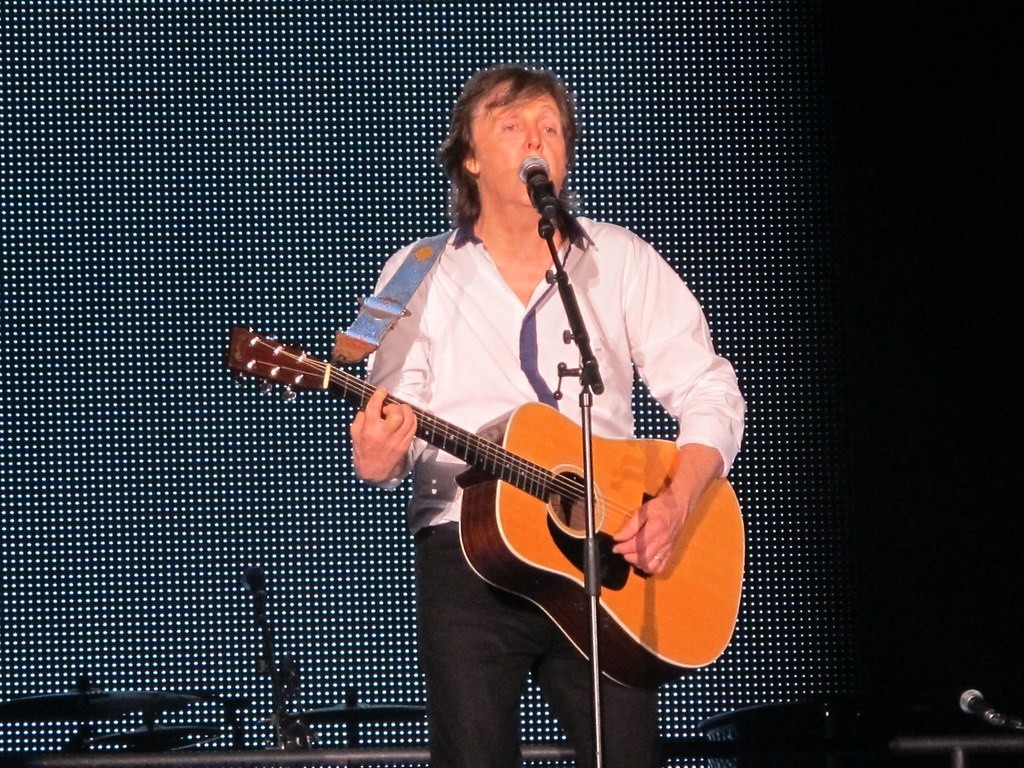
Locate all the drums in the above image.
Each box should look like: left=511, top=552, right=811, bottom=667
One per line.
left=694, top=699, right=830, bottom=768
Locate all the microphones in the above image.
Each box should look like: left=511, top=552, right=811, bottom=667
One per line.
left=241, top=567, right=267, bottom=591
left=959, top=690, right=1005, bottom=726
left=521, top=158, right=556, bottom=219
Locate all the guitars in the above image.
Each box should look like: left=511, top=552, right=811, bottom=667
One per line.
left=244, top=566, right=315, bottom=750
left=226, top=324, right=746, bottom=691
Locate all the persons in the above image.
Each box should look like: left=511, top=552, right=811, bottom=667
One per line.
left=342, top=63, right=749, bottom=768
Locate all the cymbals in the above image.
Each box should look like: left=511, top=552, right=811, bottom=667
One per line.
left=285, top=695, right=427, bottom=723
left=0, top=686, right=201, bottom=724
left=83, top=721, right=224, bottom=750
